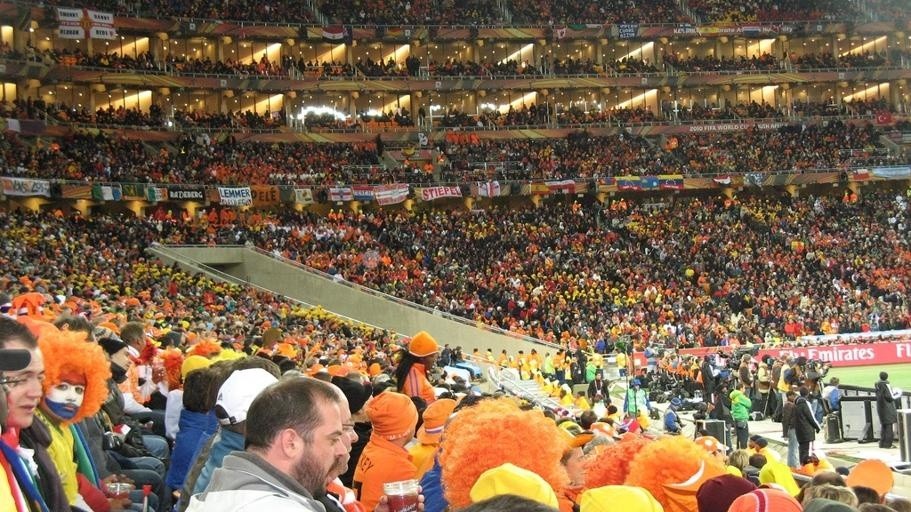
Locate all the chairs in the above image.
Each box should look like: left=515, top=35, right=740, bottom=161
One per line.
left=2, top=2, right=911, bottom=511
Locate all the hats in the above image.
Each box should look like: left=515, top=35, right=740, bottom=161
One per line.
left=845, top=459, right=894, bottom=495
left=671, top=397, right=681, bottom=404
left=559, top=427, right=595, bottom=448
left=468, top=463, right=803, bottom=512
left=181, top=355, right=211, bottom=378
left=696, top=435, right=729, bottom=454
left=94, top=327, right=128, bottom=355
left=623, top=417, right=640, bottom=433
left=417, top=398, right=457, bottom=445
left=365, top=391, right=419, bottom=441
left=215, top=367, right=280, bottom=425
left=590, top=422, right=616, bottom=439
left=750, top=434, right=767, bottom=446
left=408, top=331, right=439, bottom=357
left=631, top=378, right=640, bottom=385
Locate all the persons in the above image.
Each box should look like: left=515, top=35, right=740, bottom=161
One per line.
left=0, top=0, right=911, bottom=512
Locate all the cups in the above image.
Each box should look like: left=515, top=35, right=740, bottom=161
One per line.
left=384, top=478, right=420, bottom=511
left=153, top=357, right=165, bottom=377
left=106, top=481, right=131, bottom=501
